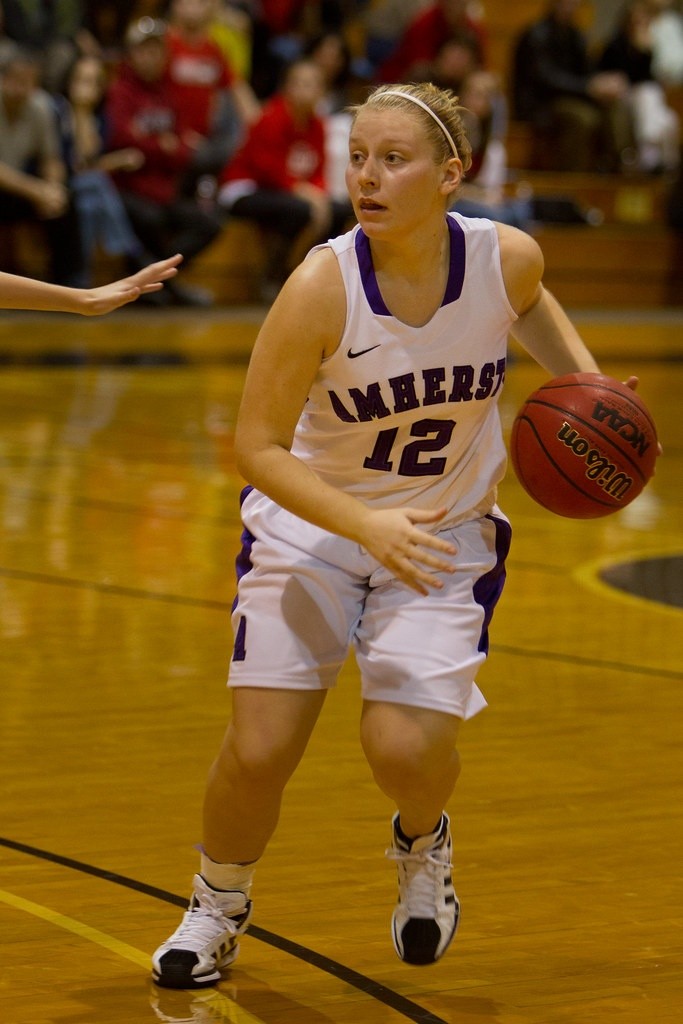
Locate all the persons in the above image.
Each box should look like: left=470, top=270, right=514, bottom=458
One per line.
left=0, top=251, right=183, bottom=317
left=1, top=0, right=682, bottom=307
left=148, top=82, right=666, bottom=993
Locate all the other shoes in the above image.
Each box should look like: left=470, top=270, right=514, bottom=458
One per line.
left=265, top=254, right=287, bottom=298
left=137, top=277, right=211, bottom=307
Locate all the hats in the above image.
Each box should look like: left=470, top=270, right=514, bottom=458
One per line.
left=127, top=20, right=171, bottom=48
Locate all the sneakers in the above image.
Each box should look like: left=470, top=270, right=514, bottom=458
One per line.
left=152, top=873, right=252, bottom=989
left=391, top=806, right=461, bottom=966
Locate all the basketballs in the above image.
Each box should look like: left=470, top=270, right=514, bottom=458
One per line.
left=510, top=373, right=657, bottom=520
